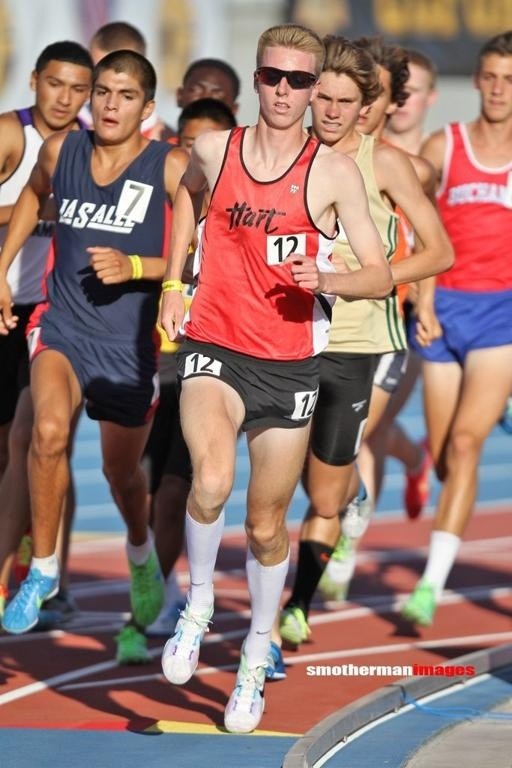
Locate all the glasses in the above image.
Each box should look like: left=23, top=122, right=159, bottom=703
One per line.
left=253, top=66, right=318, bottom=92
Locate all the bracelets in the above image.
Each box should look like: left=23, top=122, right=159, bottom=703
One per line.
left=161, top=278, right=184, bottom=294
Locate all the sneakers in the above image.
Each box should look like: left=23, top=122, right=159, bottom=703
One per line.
left=393, top=582, right=438, bottom=629
left=276, top=602, right=310, bottom=646
left=495, top=393, right=512, bottom=442
left=341, top=488, right=373, bottom=541
left=36, top=591, right=82, bottom=625
left=260, top=641, right=287, bottom=683
left=1, top=557, right=62, bottom=637
left=404, top=434, right=432, bottom=521
left=159, top=568, right=189, bottom=628
left=159, top=585, right=217, bottom=685
left=315, top=517, right=357, bottom=598
left=8, top=527, right=39, bottom=583
left=124, top=522, right=168, bottom=628
left=115, top=622, right=149, bottom=670
left=223, top=638, right=273, bottom=735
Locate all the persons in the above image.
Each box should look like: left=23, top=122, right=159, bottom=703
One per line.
left=375, top=43, right=433, bottom=530
left=160, top=26, right=395, bottom=734
left=259, top=32, right=456, bottom=683
left=399, top=30, right=512, bottom=626
left=277, top=34, right=444, bottom=645
